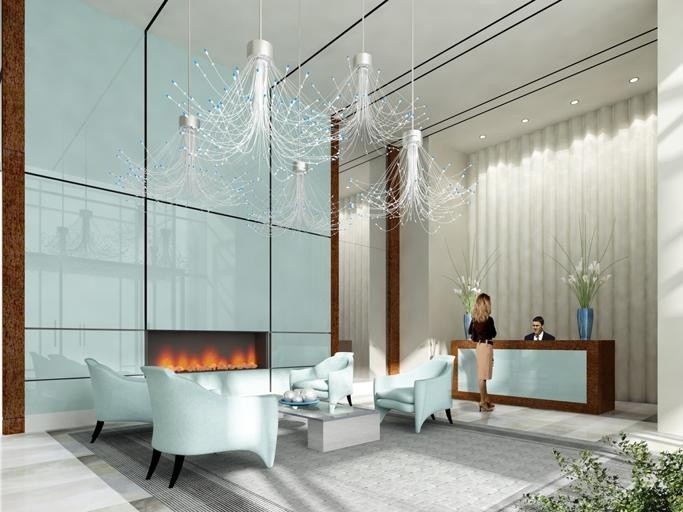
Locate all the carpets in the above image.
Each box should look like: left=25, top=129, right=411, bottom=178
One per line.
left=46, top=394, right=667, bottom=512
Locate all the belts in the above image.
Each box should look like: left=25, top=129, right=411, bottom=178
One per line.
left=476, top=339, right=493, bottom=345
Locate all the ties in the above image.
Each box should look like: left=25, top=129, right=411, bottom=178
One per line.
left=535, top=335, right=541, bottom=341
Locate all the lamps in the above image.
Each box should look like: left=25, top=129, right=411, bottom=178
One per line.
left=46, top=125, right=132, bottom=268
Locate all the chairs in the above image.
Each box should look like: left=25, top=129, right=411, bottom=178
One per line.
left=289, top=352, right=355, bottom=407
left=84, top=358, right=153, bottom=443
left=374, top=354, right=457, bottom=434
left=28, top=350, right=84, bottom=400
left=140, top=366, right=279, bottom=487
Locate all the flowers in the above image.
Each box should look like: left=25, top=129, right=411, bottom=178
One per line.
left=441, top=241, right=505, bottom=313
left=542, top=213, right=630, bottom=308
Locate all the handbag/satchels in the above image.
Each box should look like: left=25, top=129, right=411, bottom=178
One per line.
left=470, top=317, right=478, bottom=343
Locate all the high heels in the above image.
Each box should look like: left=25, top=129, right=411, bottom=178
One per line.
left=479, top=400, right=495, bottom=413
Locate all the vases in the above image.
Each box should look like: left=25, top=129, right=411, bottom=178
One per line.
left=464, top=312, right=473, bottom=341
left=576, top=307, right=593, bottom=340
